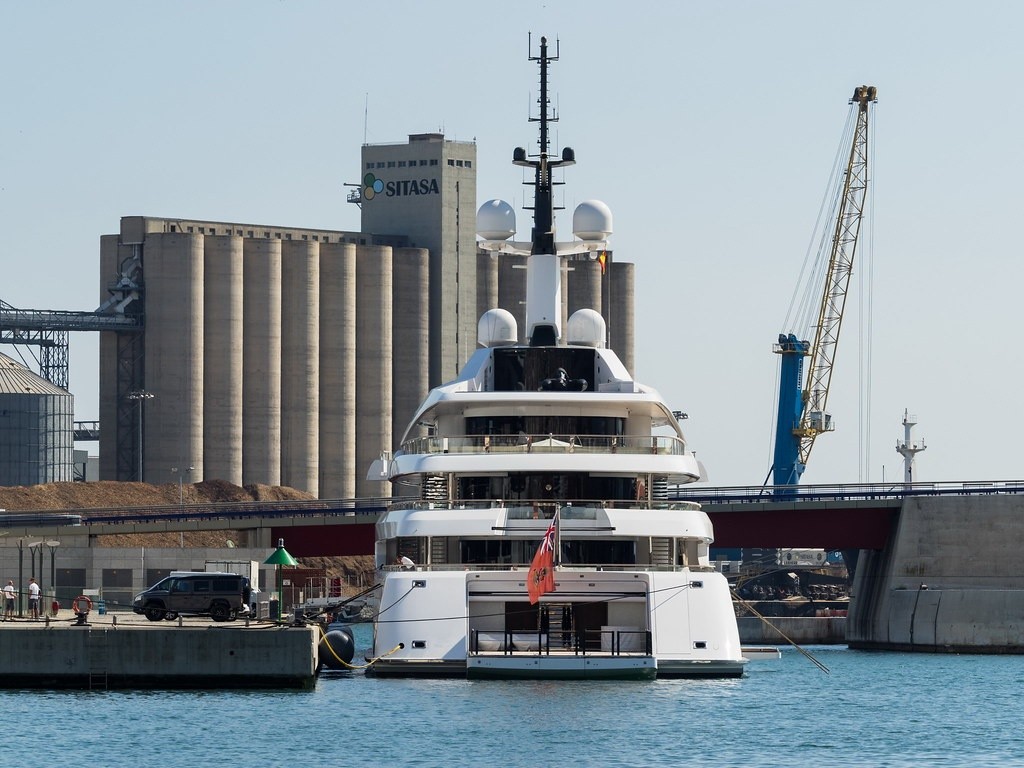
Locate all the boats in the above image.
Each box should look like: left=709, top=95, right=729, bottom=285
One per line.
left=361, top=30, right=749, bottom=682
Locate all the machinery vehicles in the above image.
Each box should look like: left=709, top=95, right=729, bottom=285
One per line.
left=735, top=83, right=880, bottom=601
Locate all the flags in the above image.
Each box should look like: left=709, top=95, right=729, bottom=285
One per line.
left=528, top=513, right=557, bottom=605
left=598, top=250, right=607, bottom=276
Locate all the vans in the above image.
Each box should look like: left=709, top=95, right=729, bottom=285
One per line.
left=132, top=573, right=245, bottom=622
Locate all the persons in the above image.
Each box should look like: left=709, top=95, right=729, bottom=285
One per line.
left=242, top=583, right=251, bottom=613
left=397, top=555, right=415, bottom=571
left=29, top=577, right=42, bottom=619
left=0, top=580, right=17, bottom=622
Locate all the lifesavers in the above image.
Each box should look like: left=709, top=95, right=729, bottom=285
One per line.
left=73, top=596, right=92, bottom=615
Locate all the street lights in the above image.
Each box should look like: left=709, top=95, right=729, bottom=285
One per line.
left=180, top=467, right=195, bottom=548
left=125, top=389, right=155, bottom=482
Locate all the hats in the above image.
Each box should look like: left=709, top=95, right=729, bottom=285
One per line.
left=8, top=581, right=12, bottom=585
left=29, top=578, right=34, bottom=581
